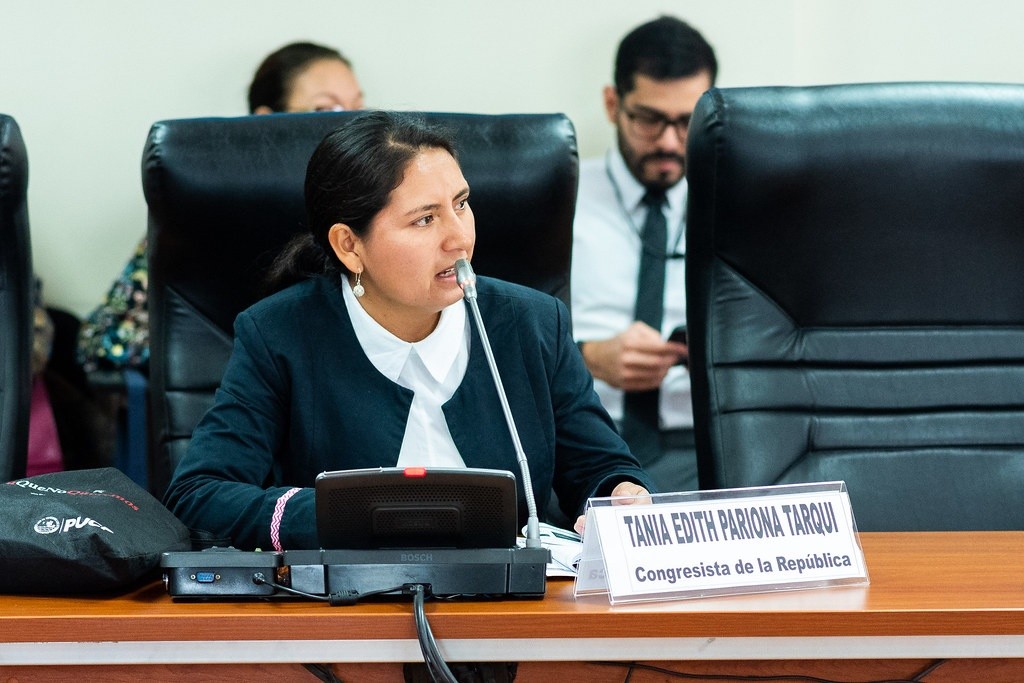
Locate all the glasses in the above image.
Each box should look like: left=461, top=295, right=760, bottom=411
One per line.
left=618, top=98, right=692, bottom=143
left=34, top=325, right=51, bottom=341
left=286, top=104, right=365, bottom=112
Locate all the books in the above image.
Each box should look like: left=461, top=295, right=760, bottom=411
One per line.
left=517, top=523, right=587, bottom=579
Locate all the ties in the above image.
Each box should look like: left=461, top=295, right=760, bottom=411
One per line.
left=620, top=191, right=669, bottom=468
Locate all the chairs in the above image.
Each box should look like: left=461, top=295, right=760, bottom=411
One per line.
left=140, top=112, right=581, bottom=525
left=0, top=112, right=34, bottom=484
left=686, top=84, right=1024, bottom=532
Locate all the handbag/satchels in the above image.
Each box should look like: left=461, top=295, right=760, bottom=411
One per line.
left=0, top=468, right=231, bottom=598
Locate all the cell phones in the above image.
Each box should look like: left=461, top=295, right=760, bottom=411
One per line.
left=665, top=326, right=688, bottom=365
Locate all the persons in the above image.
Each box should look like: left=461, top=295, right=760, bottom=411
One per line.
left=567, top=16, right=717, bottom=493
left=160, top=112, right=656, bottom=552
left=82, top=42, right=362, bottom=380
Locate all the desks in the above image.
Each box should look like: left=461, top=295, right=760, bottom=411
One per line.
left=0, top=530, right=1024, bottom=683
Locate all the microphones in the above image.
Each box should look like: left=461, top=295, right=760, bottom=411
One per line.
left=454, top=260, right=585, bottom=577
